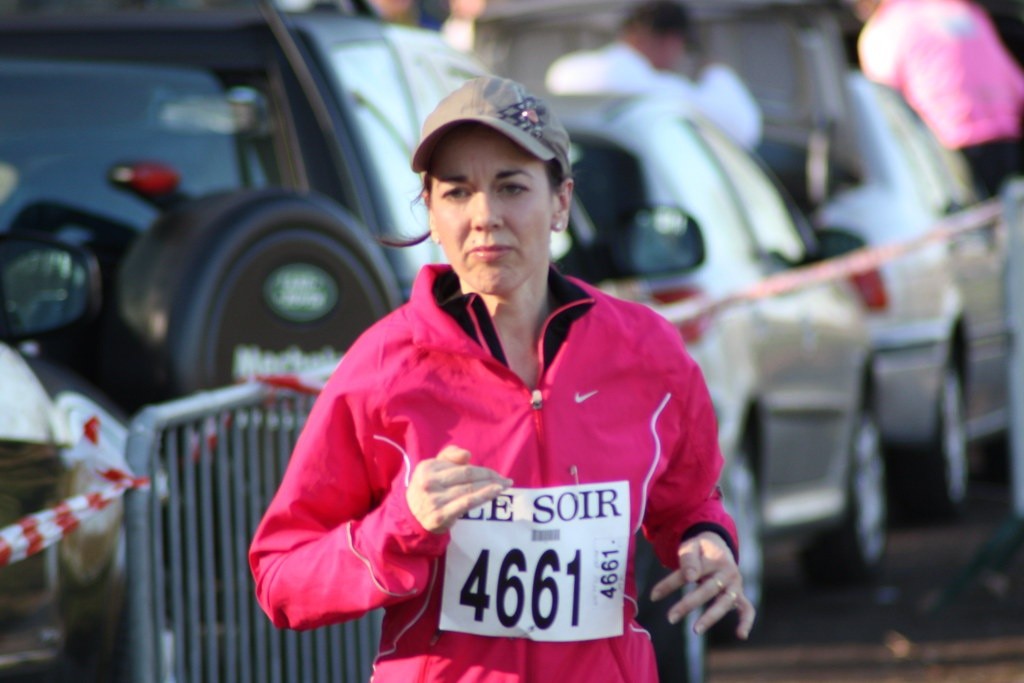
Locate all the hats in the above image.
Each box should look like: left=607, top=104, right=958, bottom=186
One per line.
left=409, top=75, right=573, bottom=178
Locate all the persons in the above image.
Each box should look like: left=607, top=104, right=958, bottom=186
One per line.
left=545, top=0, right=764, bottom=151
left=247, top=74, right=755, bottom=682
left=849, top=0, right=1023, bottom=195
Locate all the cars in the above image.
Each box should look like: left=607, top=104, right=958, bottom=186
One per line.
left=280, top=0, right=1024, bottom=651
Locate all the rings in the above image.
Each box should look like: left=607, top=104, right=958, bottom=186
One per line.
left=712, top=574, right=724, bottom=591
left=730, top=591, right=738, bottom=609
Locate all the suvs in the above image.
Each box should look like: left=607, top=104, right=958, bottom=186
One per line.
left=0, top=0, right=412, bottom=683
